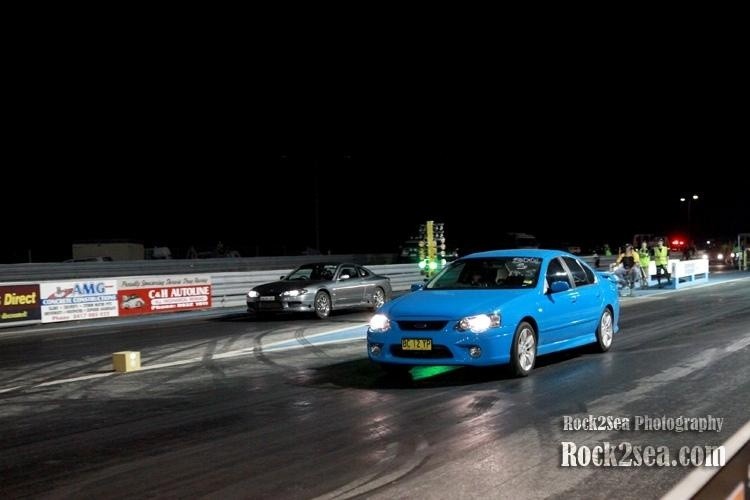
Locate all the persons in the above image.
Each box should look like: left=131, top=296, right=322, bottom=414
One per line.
left=591, top=238, right=699, bottom=292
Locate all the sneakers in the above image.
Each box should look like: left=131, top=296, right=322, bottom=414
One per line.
left=627, top=282, right=635, bottom=289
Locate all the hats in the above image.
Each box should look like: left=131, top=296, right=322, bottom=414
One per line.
left=623, top=243, right=631, bottom=248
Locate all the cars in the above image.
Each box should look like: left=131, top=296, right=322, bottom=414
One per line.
left=367, top=248, right=620, bottom=379
left=633, top=233, right=743, bottom=263
left=245, top=262, right=394, bottom=319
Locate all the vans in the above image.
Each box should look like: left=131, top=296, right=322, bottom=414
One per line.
left=568, top=246, right=581, bottom=255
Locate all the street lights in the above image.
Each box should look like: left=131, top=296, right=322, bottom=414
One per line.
left=678, top=192, right=704, bottom=222
